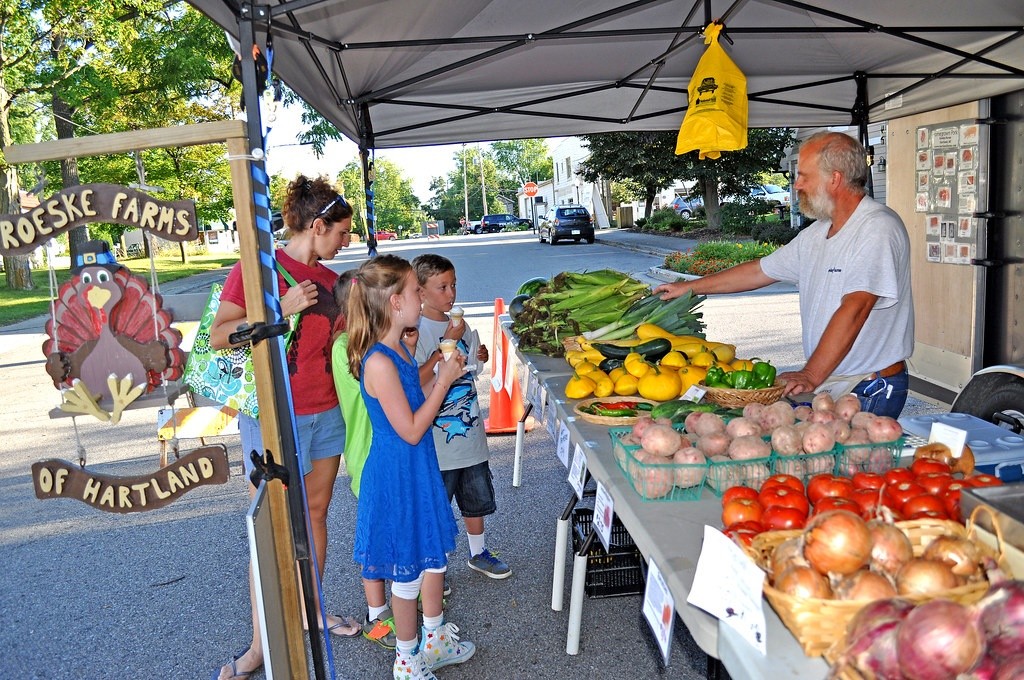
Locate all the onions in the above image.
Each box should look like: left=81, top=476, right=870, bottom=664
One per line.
left=769, top=510, right=1024, bottom=680
left=913, top=443, right=974, bottom=478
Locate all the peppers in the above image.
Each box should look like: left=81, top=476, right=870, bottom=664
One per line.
left=578, top=359, right=777, bottom=417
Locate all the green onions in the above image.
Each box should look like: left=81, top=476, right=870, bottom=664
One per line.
left=583, top=289, right=707, bottom=341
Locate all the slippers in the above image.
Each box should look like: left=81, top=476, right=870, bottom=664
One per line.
left=302, top=614, right=363, bottom=637
left=211, top=646, right=262, bottom=680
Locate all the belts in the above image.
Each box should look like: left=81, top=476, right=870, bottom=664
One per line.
left=863, top=361, right=907, bottom=381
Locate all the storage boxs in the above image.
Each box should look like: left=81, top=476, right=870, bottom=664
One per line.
left=723, top=417, right=773, bottom=441
left=609, top=427, right=642, bottom=474
left=671, top=420, right=730, bottom=448
left=896, top=412, right=1024, bottom=484
left=704, top=452, right=778, bottom=498
left=835, top=436, right=905, bottom=479
left=571, top=507, right=648, bottom=600
left=761, top=435, right=841, bottom=488
left=625, top=445, right=710, bottom=503
left=958, top=483, right=1024, bottom=553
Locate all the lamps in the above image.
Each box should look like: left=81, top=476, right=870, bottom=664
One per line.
left=788, top=171, right=794, bottom=184
left=878, top=156, right=886, bottom=173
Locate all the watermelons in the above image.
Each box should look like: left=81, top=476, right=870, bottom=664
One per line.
left=509, top=295, right=530, bottom=320
left=517, top=277, right=548, bottom=299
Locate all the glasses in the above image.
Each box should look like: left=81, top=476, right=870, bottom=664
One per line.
left=310, top=194, right=348, bottom=228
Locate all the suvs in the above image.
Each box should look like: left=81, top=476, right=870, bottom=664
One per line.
left=538, top=203, right=595, bottom=245
left=668, top=195, right=705, bottom=220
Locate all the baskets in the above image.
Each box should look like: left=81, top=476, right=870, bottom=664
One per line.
left=698, top=377, right=787, bottom=409
left=607, top=407, right=910, bottom=503
left=730, top=504, right=1012, bottom=657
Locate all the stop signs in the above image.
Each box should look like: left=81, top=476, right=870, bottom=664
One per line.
left=523, top=182, right=538, bottom=197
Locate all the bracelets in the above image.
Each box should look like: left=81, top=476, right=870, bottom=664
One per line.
left=436, top=382, right=448, bottom=391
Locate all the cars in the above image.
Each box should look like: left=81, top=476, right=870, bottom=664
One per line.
left=718, top=183, right=800, bottom=210
left=366, top=231, right=398, bottom=241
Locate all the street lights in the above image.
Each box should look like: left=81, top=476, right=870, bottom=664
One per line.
left=461, top=143, right=468, bottom=225
left=480, top=213, right=532, bottom=233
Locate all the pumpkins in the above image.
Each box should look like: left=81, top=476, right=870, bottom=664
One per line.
left=565, top=324, right=754, bottom=400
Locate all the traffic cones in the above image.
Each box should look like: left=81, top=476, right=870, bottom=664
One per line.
left=485, top=296, right=540, bottom=435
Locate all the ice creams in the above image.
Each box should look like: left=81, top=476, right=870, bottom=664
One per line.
left=449, top=305, right=465, bottom=328
left=437, top=339, right=457, bottom=362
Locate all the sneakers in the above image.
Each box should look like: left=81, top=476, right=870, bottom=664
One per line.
left=468, top=548, right=512, bottom=578
left=417, top=592, right=447, bottom=614
left=443, top=579, right=451, bottom=596
left=419, top=621, right=475, bottom=671
left=362, top=610, right=398, bottom=650
left=392, top=645, right=439, bottom=680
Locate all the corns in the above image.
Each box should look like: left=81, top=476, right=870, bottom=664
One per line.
left=510, top=270, right=652, bottom=355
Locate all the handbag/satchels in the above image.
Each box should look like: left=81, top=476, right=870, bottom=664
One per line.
left=182, top=260, right=306, bottom=418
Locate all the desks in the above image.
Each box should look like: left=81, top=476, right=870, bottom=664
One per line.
left=542, top=374, right=729, bottom=680
left=719, top=595, right=831, bottom=680
left=497, top=312, right=576, bottom=489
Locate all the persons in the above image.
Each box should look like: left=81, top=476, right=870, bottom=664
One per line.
left=346, top=254, right=475, bottom=680
left=413, top=254, right=513, bottom=597
left=651, top=131, right=916, bottom=420
left=210, top=175, right=353, bottom=680
left=331, top=269, right=410, bottom=651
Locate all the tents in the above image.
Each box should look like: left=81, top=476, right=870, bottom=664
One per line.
left=188, top=1, right=1024, bottom=680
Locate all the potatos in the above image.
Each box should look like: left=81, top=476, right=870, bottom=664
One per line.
left=615, top=393, right=903, bottom=499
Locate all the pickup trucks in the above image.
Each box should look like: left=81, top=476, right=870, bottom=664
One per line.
left=466, top=215, right=484, bottom=234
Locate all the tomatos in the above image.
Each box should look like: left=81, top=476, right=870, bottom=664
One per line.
left=721, top=457, right=1004, bottom=546
